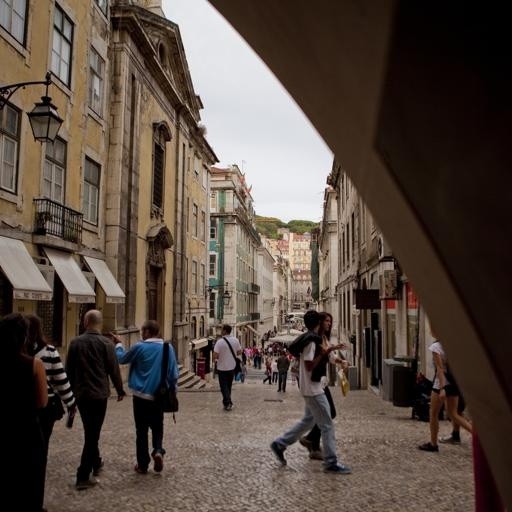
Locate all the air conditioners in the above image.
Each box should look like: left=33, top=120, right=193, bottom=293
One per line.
left=377, top=232, right=393, bottom=262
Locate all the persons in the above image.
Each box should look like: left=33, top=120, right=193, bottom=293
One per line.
left=419, top=339, right=472, bottom=451
left=212, top=324, right=241, bottom=411
left=1, top=313, right=51, bottom=512
left=270, top=309, right=351, bottom=475
left=438, top=396, right=465, bottom=445
left=109, top=318, right=178, bottom=475
left=235, top=322, right=300, bottom=393
left=299, top=312, right=347, bottom=460
left=66, top=309, right=126, bottom=490
left=24, top=314, right=77, bottom=429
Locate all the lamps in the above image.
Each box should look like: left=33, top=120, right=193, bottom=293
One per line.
left=0, top=72, right=63, bottom=145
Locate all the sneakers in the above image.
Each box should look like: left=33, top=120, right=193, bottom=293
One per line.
left=309, top=450, right=321, bottom=459
left=76, top=480, right=95, bottom=490
left=418, top=442, right=438, bottom=452
left=438, top=435, right=460, bottom=444
left=153, top=453, right=163, bottom=472
left=135, top=464, right=146, bottom=473
left=94, top=462, right=104, bottom=475
left=321, top=462, right=351, bottom=474
left=300, top=438, right=312, bottom=451
left=271, top=441, right=287, bottom=465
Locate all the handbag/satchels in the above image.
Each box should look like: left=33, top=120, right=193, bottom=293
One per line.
left=235, top=358, right=242, bottom=381
left=338, top=369, right=350, bottom=396
left=52, top=395, right=65, bottom=421
left=156, top=380, right=177, bottom=412
